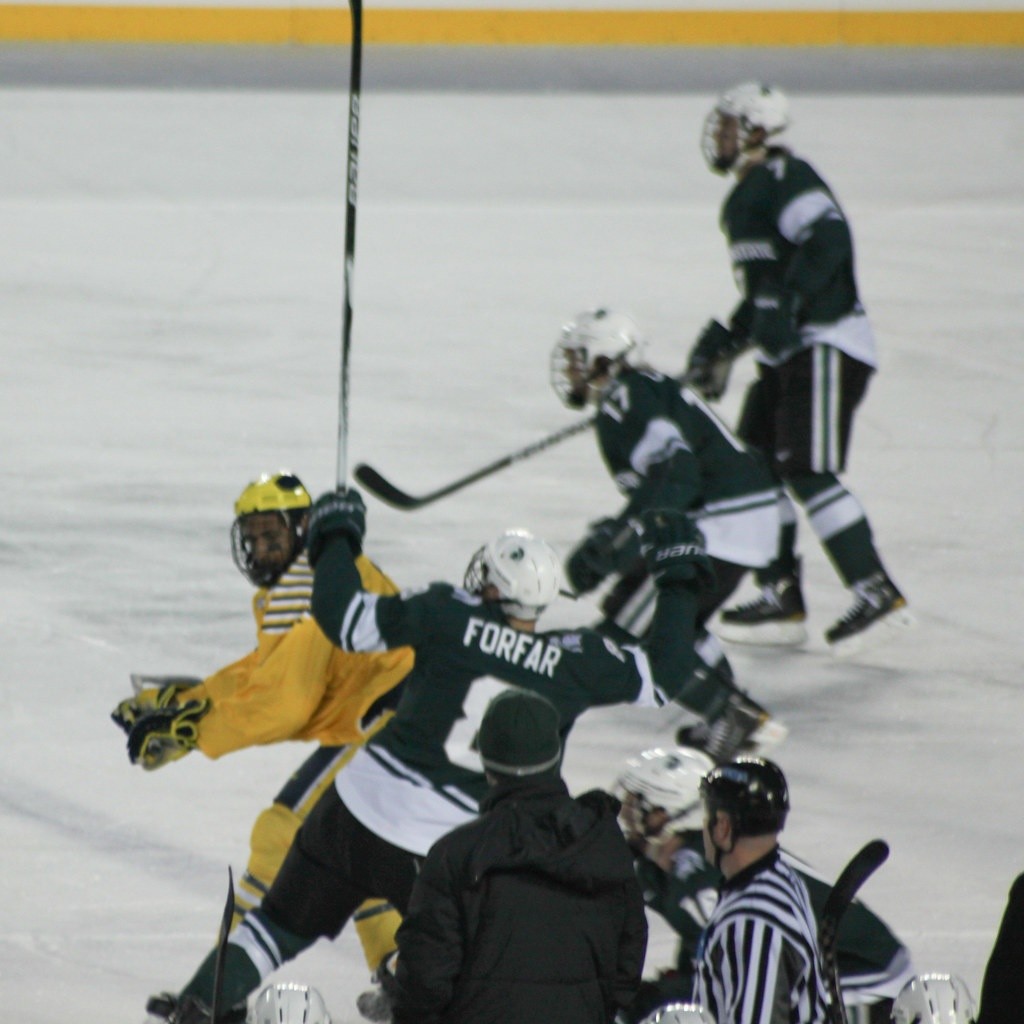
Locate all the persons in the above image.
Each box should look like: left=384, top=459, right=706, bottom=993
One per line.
left=551, top=307, right=788, bottom=762
left=112, top=471, right=415, bottom=1023
left=379, top=688, right=648, bottom=1024
left=689, top=81, right=909, bottom=645
left=175, top=491, right=654, bottom=1023
left=690, top=755, right=826, bottom=1024
left=613, top=748, right=916, bottom=1006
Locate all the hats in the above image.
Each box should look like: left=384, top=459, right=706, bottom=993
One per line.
left=479, top=688, right=563, bottom=781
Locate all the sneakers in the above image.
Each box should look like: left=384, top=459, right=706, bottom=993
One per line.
left=676, top=717, right=715, bottom=749
left=719, top=555, right=807, bottom=645
left=356, top=950, right=400, bottom=1024
left=145, top=991, right=248, bottom=1024
left=703, top=690, right=790, bottom=759
left=825, top=568, right=913, bottom=656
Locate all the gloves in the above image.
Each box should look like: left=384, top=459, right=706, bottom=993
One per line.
left=567, top=516, right=638, bottom=592
left=618, top=970, right=677, bottom=1024
left=307, top=488, right=366, bottom=565
left=640, top=508, right=718, bottom=591
left=752, top=283, right=803, bottom=362
left=688, top=320, right=735, bottom=399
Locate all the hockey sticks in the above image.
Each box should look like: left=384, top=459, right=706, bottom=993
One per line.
left=354, top=367, right=689, bottom=512
left=817, top=839, right=891, bottom=1024
left=336, top=0, right=362, bottom=496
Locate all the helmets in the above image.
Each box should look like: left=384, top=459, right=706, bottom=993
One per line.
left=246, top=982, right=333, bottom=1024
left=701, top=85, right=789, bottom=174
left=889, top=973, right=978, bottom=1024
left=464, top=530, right=560, bottom=621
left=612, top=745, right=715, bottom=854
left=639, top=1003, right=713, bottom=1024
left=230, top=472, right=312, bottom=588
left=548, top=311, right=634, bottom=409
left=698, top=756, right=790, bottom=830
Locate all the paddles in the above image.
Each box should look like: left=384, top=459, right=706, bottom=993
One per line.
left=210, top=865, right=235, bottom=1024
